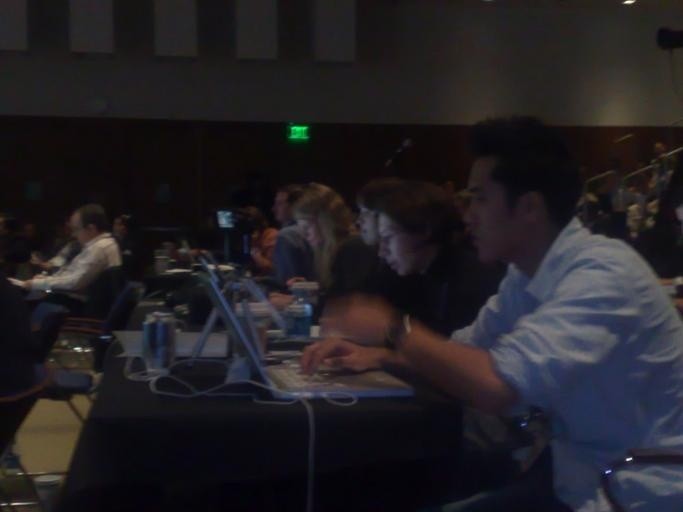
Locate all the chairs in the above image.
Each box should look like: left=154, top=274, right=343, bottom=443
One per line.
left=513, top=411, right=683, bottom=511
left=0, top=286, right=93, bottom=512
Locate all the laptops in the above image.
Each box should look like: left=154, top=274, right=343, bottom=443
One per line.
left=198, top=273, right=416, bottom=402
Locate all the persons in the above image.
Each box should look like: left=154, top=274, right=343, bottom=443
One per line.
left=1, top=140, right=505, bottom=338
left=296, top=112, right=682, bottom=511
left=576, top=141, right=683, bottom=285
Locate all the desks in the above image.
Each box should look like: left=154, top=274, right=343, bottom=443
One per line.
left=143, top=251, right=243, bottom=292
left=93, top=300, right=427, bottom=511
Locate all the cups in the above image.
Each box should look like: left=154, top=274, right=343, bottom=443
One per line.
left=233, top=302, right=270, bottom=358
left=292, top=280, right=320, bottom=324
left=142, top=311, right=186, bottom=372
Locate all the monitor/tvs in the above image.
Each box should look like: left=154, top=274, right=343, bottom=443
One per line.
left=214, top=209, right=236, bottom=230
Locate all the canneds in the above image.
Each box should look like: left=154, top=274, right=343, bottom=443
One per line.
left=142, top=312, right=175, bottom=371
